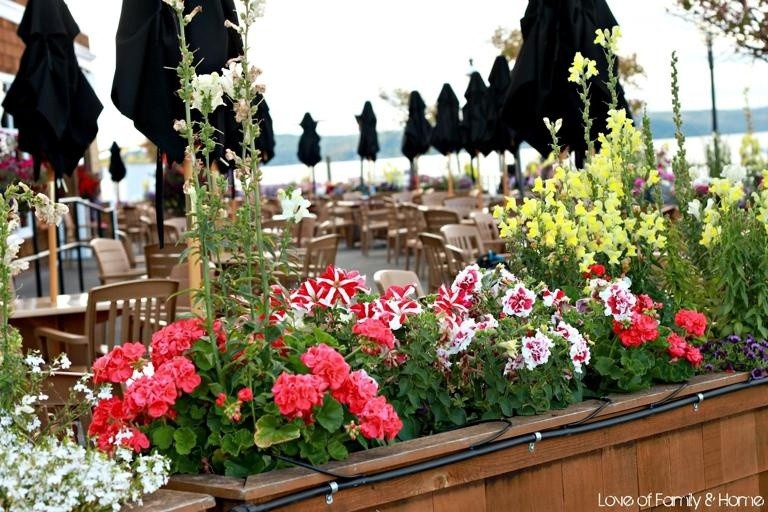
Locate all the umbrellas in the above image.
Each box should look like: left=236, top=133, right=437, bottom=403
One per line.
left=353, top=100, right=380, bottom=190
left=0, top=0, right=107, bottom=305
left=241, top=89, right=276, bottom=165
left=399, top=89, right=436, bottom=191
left=109, top=0, right=277, bottom=313
left=105, top=141, right=127, bottom=208
left=496, top=0, right=637, bottom=168
left=432, top=82, right=463, bottom=200
left=457, top=70, right=494, bottom=217
left=486, top=55, right=524, bottom=211
left=294, top=113, right=321, bottom=195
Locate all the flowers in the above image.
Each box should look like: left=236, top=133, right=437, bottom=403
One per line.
left=87, top=1, right=768, bottom=512
left=0, top=181, right=172, bottom=512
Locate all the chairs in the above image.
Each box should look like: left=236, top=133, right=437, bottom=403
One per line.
left=26, top=193, right=685, bottom=439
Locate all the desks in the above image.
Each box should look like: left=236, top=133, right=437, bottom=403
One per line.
left=9, top=292, right=157, bottom=359
